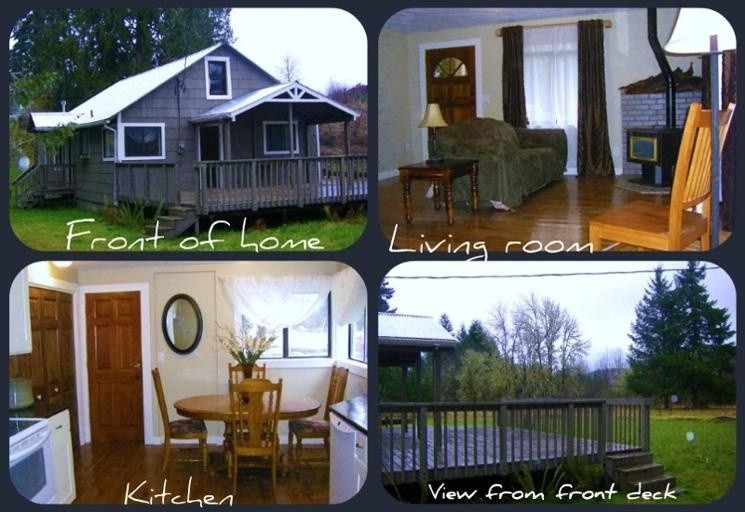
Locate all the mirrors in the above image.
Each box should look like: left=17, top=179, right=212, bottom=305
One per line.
left=161, top=293, right=203, bottom=355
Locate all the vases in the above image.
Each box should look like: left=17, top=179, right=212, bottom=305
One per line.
left=241, top=364, right=254, bottom=404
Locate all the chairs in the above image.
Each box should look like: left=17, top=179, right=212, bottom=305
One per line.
left=225, top=378, right=283, bottom=498
left=151, top=366, right=210, bottom=473
left=225, top=361, right=266, bottom=472
left=288, top=364, right=350, bottom=480
left=588, top=101, right=736, bottom=252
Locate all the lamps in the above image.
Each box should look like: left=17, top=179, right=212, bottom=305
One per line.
left=664, top=7, right=737, bottom=252
left=417, top=102, right=449, bottom=164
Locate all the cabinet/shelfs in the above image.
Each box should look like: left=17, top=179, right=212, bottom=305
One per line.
left=47, top=409, right=77, bottom=505
left=354, top=429, right=368, bottom=492
left=8, top=267, right=79, bottom=453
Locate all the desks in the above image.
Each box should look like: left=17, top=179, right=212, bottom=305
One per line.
left=398, top=159, right=480, bottom=226
left=173, top=393, right=321, bottom=477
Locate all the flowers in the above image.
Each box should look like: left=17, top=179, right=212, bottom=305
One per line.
left=212, top=314, right=279, bottom=365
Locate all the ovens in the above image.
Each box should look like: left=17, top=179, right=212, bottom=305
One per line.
left=9, top=425, right=56, bottom=504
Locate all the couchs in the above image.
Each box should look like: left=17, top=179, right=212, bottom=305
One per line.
left=428, top=117, right=568, bottom=210
